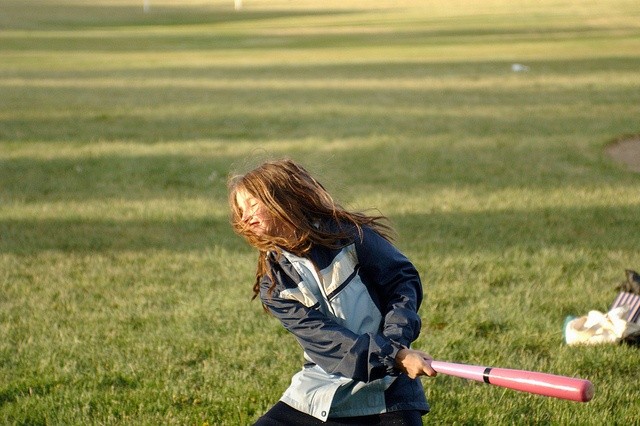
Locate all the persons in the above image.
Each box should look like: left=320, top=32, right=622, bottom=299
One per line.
left=228, top=158, right=438, bottom=426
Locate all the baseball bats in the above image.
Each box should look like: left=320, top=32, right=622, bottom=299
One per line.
left=423, top=358, right=595, bottom=403
